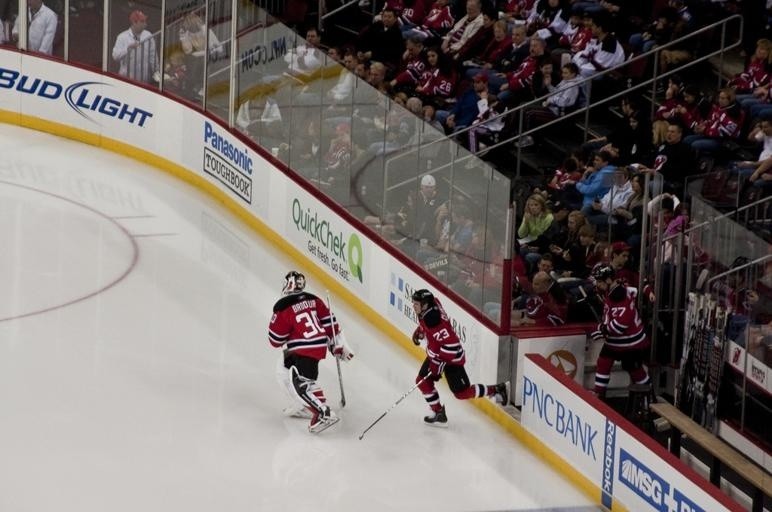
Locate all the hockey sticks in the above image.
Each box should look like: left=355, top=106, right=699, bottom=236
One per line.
left=326, top=290, right=346, bottom=412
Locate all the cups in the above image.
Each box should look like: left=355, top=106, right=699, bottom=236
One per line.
left=420, top=238, right=428, bottom=248
left=272, top=148, right=279, bottom=157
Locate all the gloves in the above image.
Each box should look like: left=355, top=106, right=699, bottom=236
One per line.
left=332, top=344, right=355, bottom=362
left=428, top=356, right=447, bottom=380
left=412, top=327, right=424, bottom=346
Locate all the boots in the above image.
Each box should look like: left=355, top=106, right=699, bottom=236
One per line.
left=309, top=403, right=335, bottom=428
left=490, top=382, right=508, bottom=407
left=289, top=395, right=328, bottom=418
left=424, top=404, right=448, bottom=425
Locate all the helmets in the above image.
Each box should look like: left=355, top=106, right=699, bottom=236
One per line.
left=593, top=264, right=617, bottom=282
left=282, top=271, right=306, bottom=294
left=411, top=288, right=435, bottom=311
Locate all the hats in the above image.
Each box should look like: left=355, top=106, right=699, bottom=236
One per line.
left=128, top=10, right=148, bottom=23
left=421, top=174, right=437, bottom=187
left=612, top=241, right=631, bottom=252
